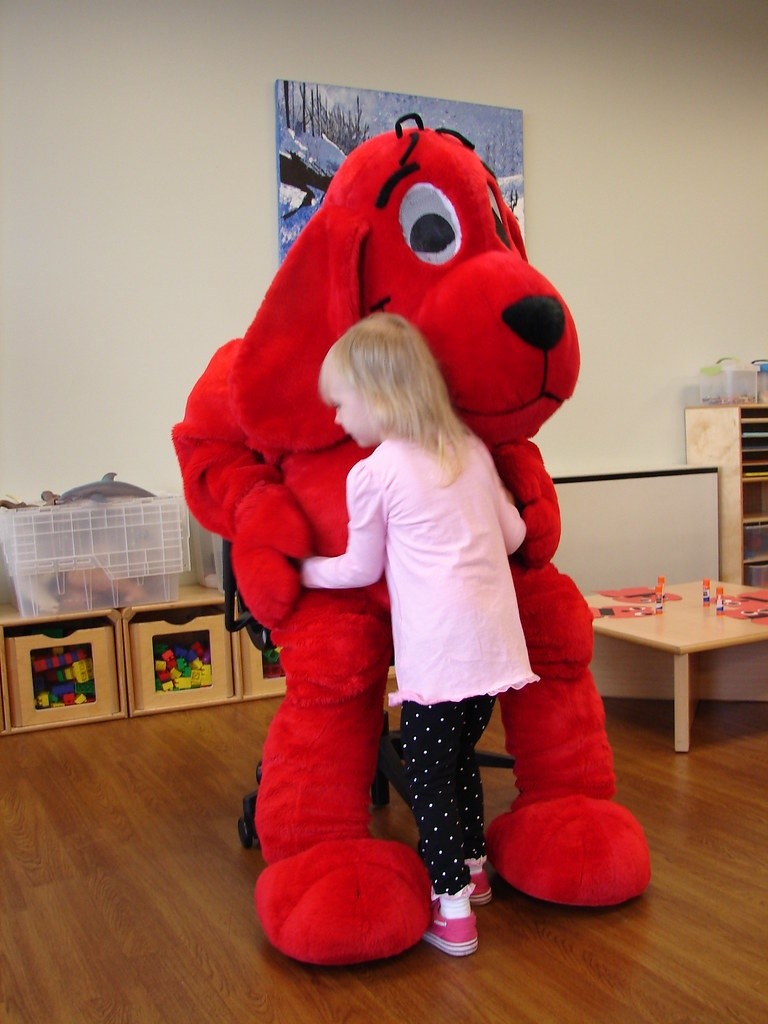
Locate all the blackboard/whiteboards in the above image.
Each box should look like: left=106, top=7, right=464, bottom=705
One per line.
left=548, top=466, right=719, bottom=706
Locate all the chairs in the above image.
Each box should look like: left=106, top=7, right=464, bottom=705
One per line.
left=220, top=533, right=516, bottom=849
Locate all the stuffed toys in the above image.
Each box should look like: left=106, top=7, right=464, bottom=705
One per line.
left=172, top=112, right=650, bottom=966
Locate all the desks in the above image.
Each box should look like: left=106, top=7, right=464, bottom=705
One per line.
left=584, top=580, right=767, bottom=752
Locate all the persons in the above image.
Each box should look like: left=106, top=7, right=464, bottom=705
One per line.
left=290, top=313, right=539, bottom=956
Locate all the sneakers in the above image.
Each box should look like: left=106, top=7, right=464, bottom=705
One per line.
left=421, top=901, right=478, bottom=958
left=469, top=870, right=493, bottom=905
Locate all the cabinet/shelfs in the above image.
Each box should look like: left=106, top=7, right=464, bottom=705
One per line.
left=683, top=404, right=768, bottom=589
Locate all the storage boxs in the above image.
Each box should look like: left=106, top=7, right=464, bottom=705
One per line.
left=128, top=607, right=234, bottom=710
left=3, top=618, right=119, bottom=727
left=698, top=362, right=758, bottom=405
left=1, top=492, right=190, bottom=617
left=755, top=363, right=767, bottom=405
left=238, top=610, right=287, bottom=695
left=188, top=505, right=228, bottom=595
